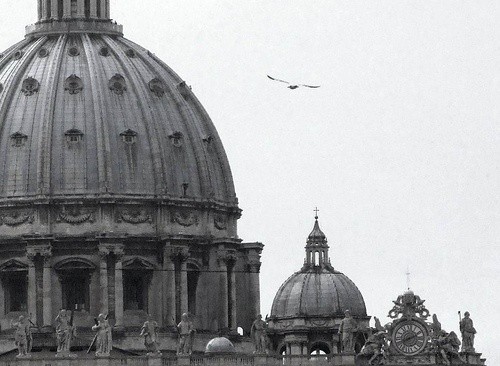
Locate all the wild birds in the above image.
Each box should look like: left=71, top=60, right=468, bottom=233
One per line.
left=267, top=75, right=320, bottom=90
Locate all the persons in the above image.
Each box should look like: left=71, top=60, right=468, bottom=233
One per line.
left=14, top=301, right=478, bottom=359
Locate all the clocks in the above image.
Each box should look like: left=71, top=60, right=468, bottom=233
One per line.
left=392, top=320, right=428, bottom=356
